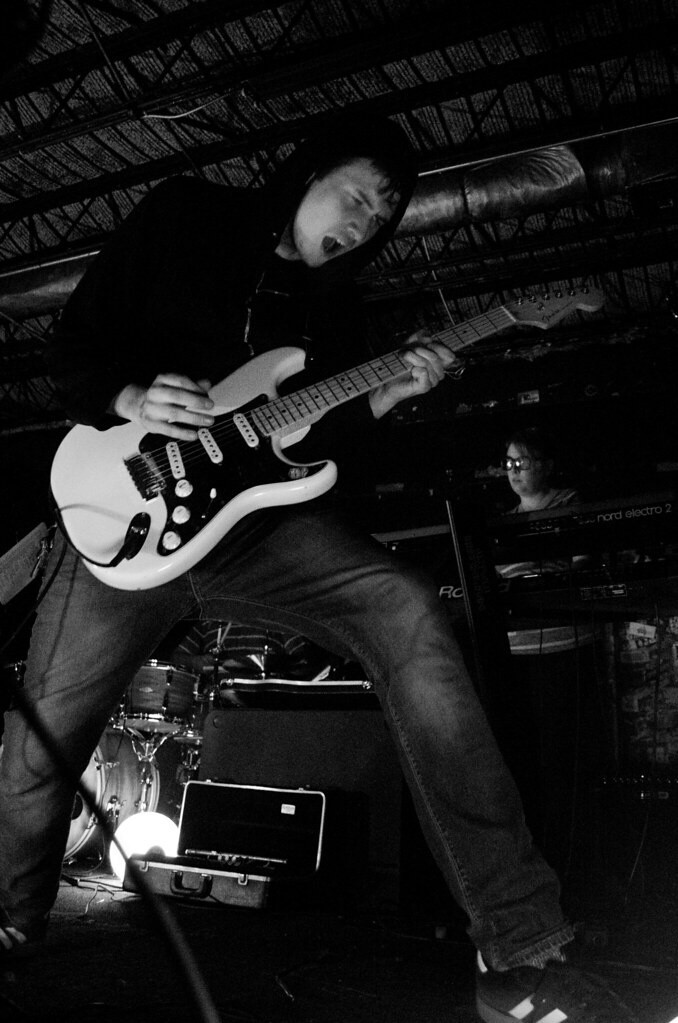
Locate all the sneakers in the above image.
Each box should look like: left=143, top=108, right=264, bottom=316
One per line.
left=475, top=950, right=639, bottom=1023
left=0, top=910, right=50, bottom=957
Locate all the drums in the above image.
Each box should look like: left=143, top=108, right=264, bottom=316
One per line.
left=116, top=658, right=205, bottom=737
left=61, top=727, right=166, bottom=872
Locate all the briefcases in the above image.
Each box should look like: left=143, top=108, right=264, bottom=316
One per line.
left=122, top=778, right=326, bottom=911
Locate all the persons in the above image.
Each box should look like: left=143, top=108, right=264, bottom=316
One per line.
left=496, top=432, right=645, bottom=930
left=174, top=617, right=309, bottom=679
left=0, top=116, right=637, bottom=1023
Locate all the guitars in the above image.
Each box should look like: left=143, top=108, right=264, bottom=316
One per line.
left=43, top=281, right=613, bottom=595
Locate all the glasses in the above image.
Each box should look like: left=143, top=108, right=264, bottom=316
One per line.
left=499, top=455, right=532, bottom=470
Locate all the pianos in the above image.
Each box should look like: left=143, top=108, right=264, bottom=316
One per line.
left=480, top=570, right=678, bottom=619
left=485, top=488, right=678, bottom=550
